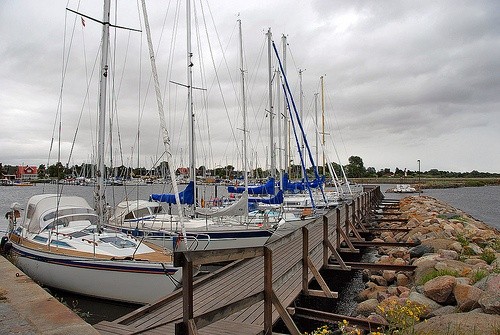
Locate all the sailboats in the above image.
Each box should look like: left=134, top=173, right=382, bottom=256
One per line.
left=0, top=0, right=366, bottom=305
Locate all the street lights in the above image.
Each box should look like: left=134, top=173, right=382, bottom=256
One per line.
left=417, top=160, right=421, bottom=196
